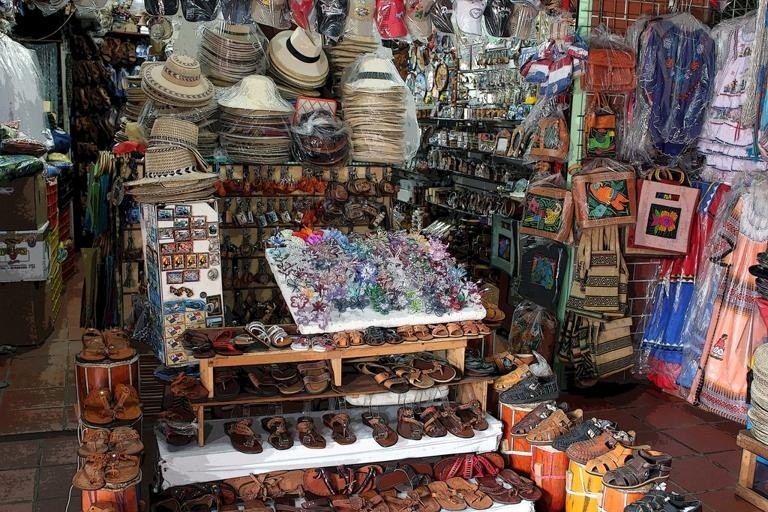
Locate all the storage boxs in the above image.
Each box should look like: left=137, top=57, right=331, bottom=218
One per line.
left=0, top=280, right=58, bottom=348
left=0, top=227, right=50, bottom=283
left=0, top=167, right=48, bottom=230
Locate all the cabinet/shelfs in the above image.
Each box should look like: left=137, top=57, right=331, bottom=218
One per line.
left=185, top=331, right=495, bottom=448
left=422, top=117, right=518, bottom=219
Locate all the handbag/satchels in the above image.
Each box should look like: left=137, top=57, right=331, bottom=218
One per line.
left=507, top=8, right=639, bottom=397
left=78, top=150, right=121, bottom=331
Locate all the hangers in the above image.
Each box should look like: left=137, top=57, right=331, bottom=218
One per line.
left=636, top=0, right=707, bottom=42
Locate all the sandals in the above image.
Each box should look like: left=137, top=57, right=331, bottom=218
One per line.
left=71, top=327, right=144, bottom=490
left=495, top=353, right=702, bottom=512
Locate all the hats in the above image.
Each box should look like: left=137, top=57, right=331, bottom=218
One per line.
left=144, top=0, right=539, bottom=39
left=121, top=22, right=406, bottom=204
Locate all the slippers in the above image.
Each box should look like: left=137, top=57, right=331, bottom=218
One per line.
left=148, top=454, right=542, bottom=512
left=159, top=305, right=505, bottom=454
left=70, top=35, right=136, bottom=131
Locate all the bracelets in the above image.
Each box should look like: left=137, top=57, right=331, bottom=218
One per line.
left=474, top=41, right=525, bottom=107
left=448, top=190, right=515, bottom=216
